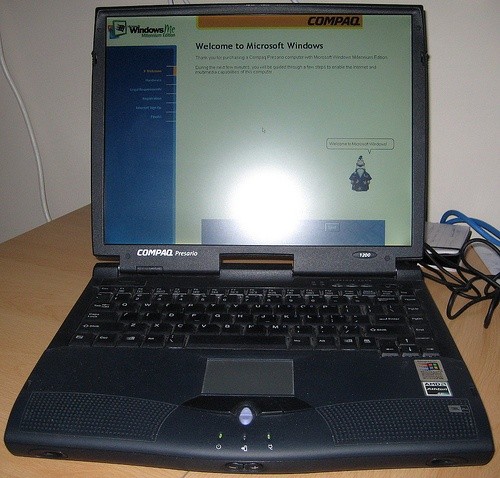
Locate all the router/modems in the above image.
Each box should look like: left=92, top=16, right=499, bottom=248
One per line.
left=424, top=221, right=474, bottom=268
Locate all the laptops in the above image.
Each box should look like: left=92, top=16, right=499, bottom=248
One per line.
left=3, top=6, right=495, bottom=475
